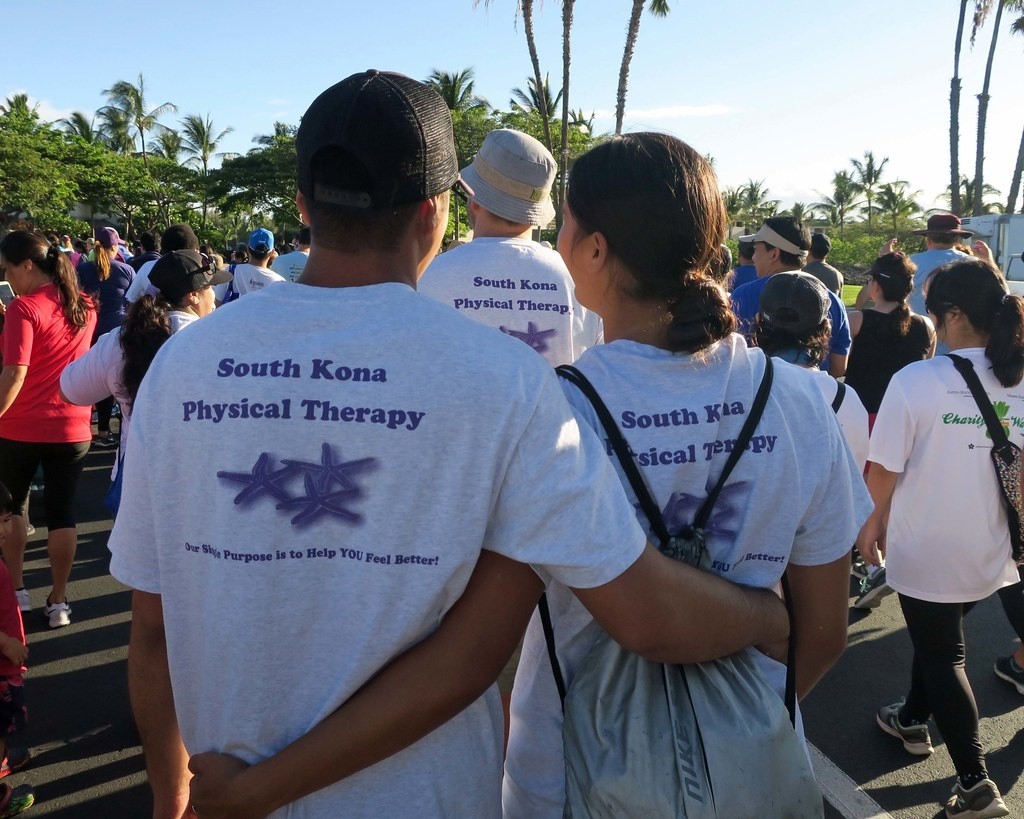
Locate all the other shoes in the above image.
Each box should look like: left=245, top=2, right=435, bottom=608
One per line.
left=112, top=405, right=121, bottom=416
left=91, top=411, right=99, bottom=424
left=1, top=747, right=30, bottom=780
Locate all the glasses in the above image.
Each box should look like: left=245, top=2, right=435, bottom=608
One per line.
left=178, top=252, right=217, bottom=286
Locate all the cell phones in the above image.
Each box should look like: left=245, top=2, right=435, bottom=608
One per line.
left=0, top=280, right=15, bottom=309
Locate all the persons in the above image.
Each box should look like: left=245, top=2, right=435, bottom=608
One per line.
left=0, top=216, right=1024, bottom=815
left=189, top=133, right=876, bottom=819
left=862, top=262, right=1024, bottom=819
left=107, top=70, right=790, bottom=819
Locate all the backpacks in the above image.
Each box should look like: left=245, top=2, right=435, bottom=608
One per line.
left=536, top=355, right=825, bottom=819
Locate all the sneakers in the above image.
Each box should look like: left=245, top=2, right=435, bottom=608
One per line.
left=945, top=778, right=1009, bottom=819
left=15, top=589, right=32, bottom=612
left=851, top=562, right=868, bottom=579
left=993, top=655, right=1023, bottom=695
left=44, top=595, right=71, bottom=627
left=855, top=569, right=895, bottom=609
left=93, top=434, right=120, bottom=448
left=876, top=696, right=932, bottom=755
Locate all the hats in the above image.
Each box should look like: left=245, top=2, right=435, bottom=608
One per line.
left=863, top=253, right=908, bottom=279
left=912, top=212, right=973, bottom=239
left=457, top=129, right=558, bottom=226
left=98, top=227, right=126, bottom=247
left=758, top=269, right=832, bottom=330
left=148, top=249, right=233, bottom=297
left=738, top=223, right=809, bottom=257
left=297, top=69, right=475, bottom=209
left=249, top=228, right=274, bottom=253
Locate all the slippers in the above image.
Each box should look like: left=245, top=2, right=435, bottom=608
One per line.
left=0, top=782, right=35, bottom=818
